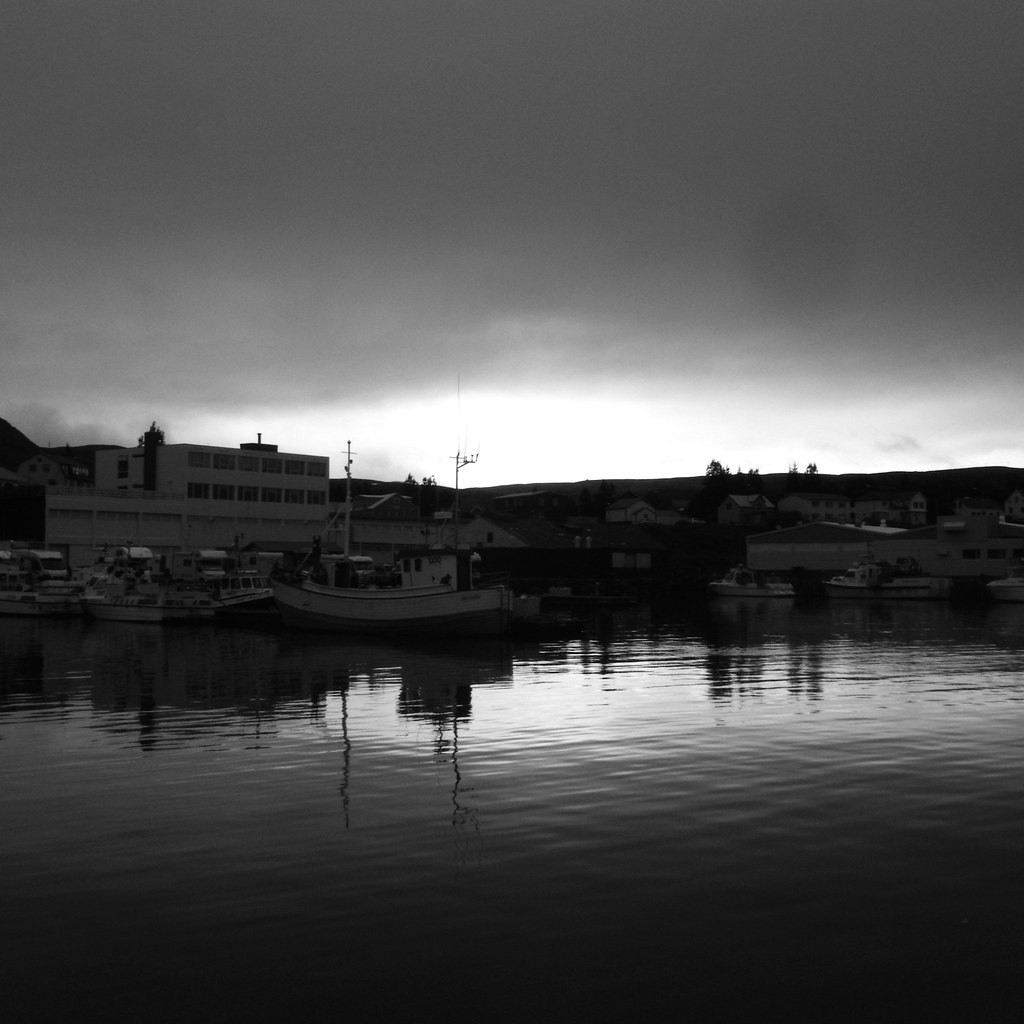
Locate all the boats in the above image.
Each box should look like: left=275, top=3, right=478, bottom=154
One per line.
left=986, top=564, right=1024, bottom=602
left=821, top=533, right=956, bottom=600
left=170, top=548, right=275, bottom=609
left=0, top=539, right=85, bottom=616
left=707, top=561, right=797, bottom=598
left=75, top=534, right=218, bottom=625
left=264, top=441, right=517, bottom=640
